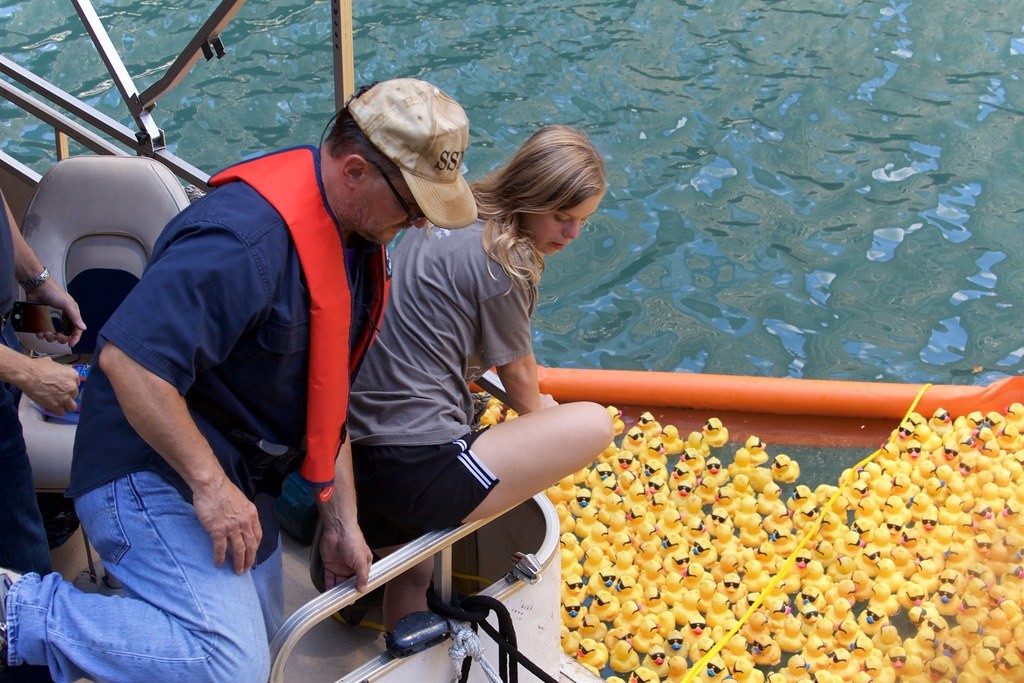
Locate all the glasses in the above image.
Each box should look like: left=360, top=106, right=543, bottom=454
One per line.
left=363, top=155, right=427, bottom=222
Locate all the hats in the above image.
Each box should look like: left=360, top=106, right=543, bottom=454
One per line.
left=346, top=77, right=479, bottom=230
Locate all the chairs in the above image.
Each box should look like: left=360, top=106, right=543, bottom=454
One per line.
left=11, top=154, right=195, bottom=492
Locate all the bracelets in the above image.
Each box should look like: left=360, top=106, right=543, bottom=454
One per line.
left=18, top=266, right=51, bottom=290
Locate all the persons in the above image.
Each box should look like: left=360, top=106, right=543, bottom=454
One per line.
left=0, top=78, right=481, bottom=683
left=0, top=184, right=88, bottom=683
left=311, top=127, right=615, bottom=654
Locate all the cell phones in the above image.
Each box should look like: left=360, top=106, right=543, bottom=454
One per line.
left=10, top=300, right=74, bottom=336
